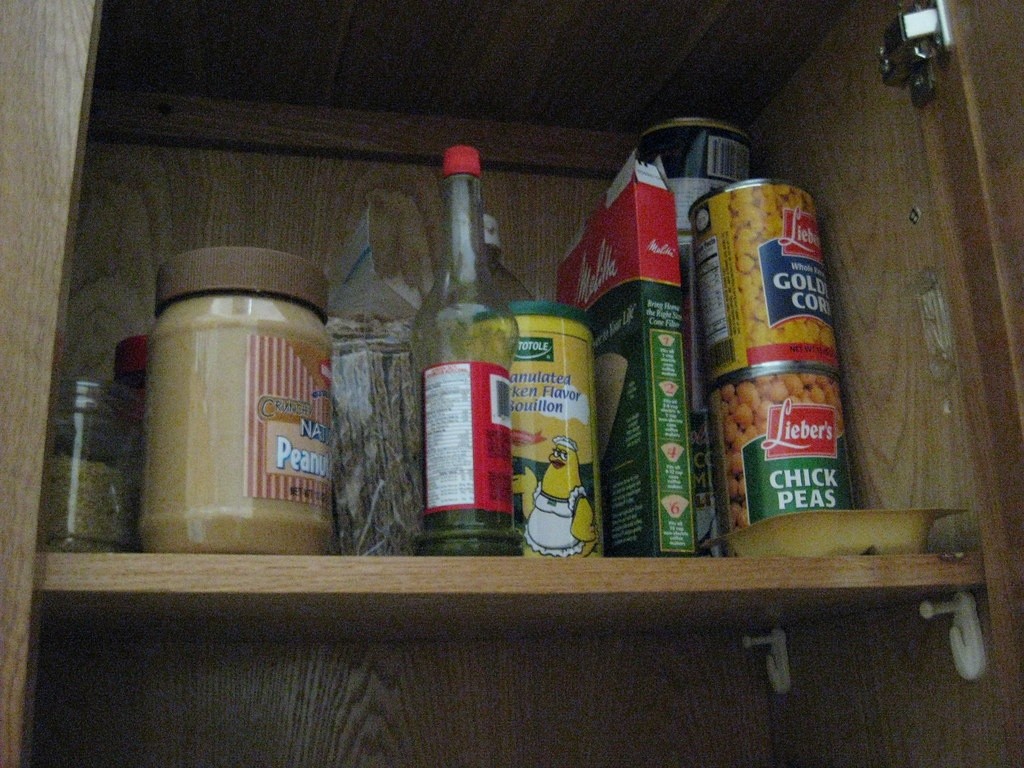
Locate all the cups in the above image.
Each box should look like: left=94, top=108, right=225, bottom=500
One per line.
left=36, top=380, right=145, bottom=553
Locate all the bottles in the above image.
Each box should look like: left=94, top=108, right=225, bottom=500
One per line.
left=484, top=215, right=533, bottom=303
left=408, top=145, right=519, bottom=557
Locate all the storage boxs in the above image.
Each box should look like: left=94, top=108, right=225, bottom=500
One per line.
left=554, top=148, right=696, bottom=557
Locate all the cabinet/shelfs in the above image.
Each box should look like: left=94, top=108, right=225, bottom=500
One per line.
left=0, top=0, right=1024, bottom=768
